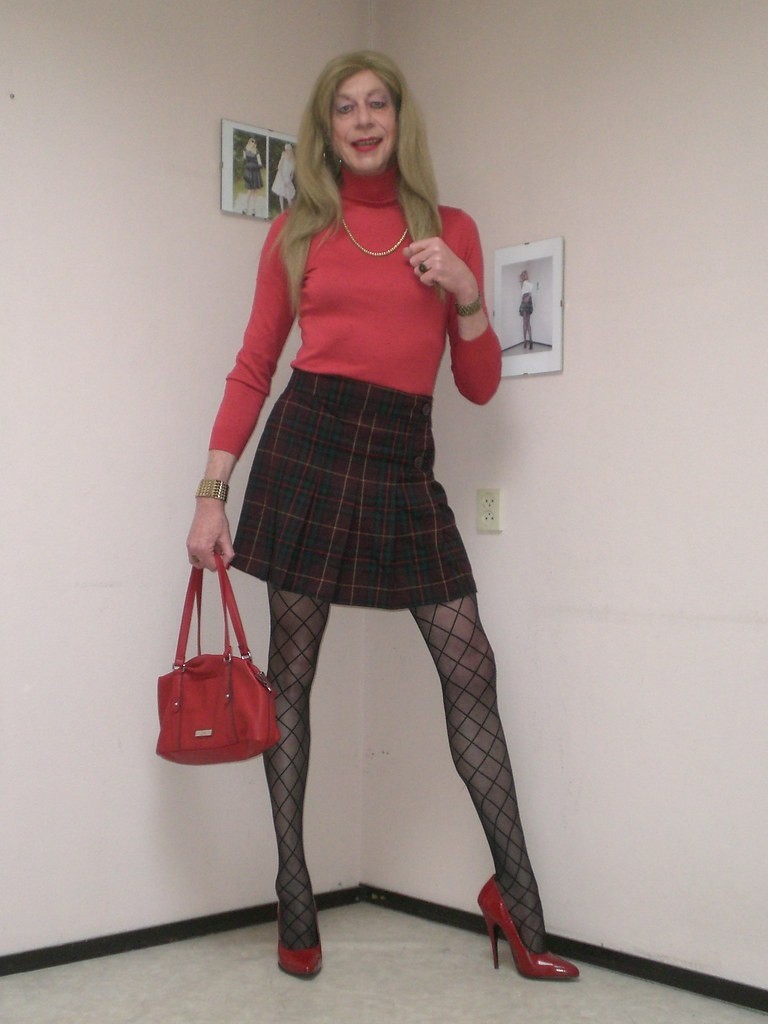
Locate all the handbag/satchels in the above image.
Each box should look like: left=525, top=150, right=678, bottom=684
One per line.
left=156, top=546, right=282, bottom=765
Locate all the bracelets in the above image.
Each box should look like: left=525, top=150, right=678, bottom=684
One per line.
left=455, top=295, right=481, bottom=316
left=195, top=479, right=229, bottom=503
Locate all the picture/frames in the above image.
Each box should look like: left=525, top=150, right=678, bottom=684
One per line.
left=494, top=236, right=564, bottom=377
left=221, top=120, right=304, bottom=220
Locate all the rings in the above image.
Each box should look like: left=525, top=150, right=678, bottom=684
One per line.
left=419, top=263, right=427, bottom=273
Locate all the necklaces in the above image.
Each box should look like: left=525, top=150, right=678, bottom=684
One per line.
left=342, top=218, right=408, bottom=257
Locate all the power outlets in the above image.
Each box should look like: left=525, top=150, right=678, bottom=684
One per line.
left=477, top=487, right=504, bottom=532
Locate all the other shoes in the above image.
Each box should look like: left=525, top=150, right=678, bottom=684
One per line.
left=242, top=210, right=246, bottom=214
left=252, top=213, right=255, bottom=216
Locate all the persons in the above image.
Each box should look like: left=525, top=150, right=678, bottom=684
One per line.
left=519, top=270, right=533, bottom=349
left=278, top=144, right=296, bottom=213
left=242, top=138, right=263, bottom=216
left=186, top=50, right=580, bottom=981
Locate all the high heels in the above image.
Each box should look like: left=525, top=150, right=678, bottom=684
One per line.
left=477, top=874, right=579, bottom=979
left=524, top=340, right=533, bottom=349
left=277, top=897, right=322, bottom=975
